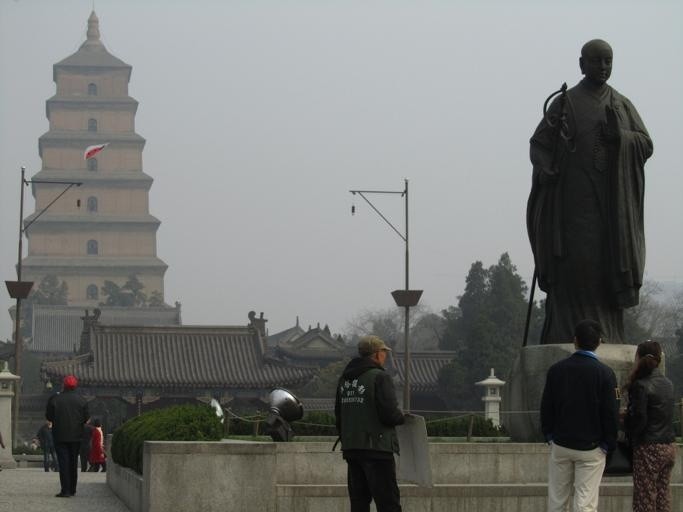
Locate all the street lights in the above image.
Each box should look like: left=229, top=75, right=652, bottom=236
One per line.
left=347, top=178, right=415, bottom=416
left=13, top=158, right=82, bottom=454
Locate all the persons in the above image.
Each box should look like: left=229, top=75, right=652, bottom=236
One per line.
left=89, top=418, right=108, bottom=472
left=334, top=335, right=407, bottom=511
left=621, top=339, right=676, bottom=511
left=44, top=374, right=89, bottom=497
left=538, top=320, right=618, bottom=512
left=527, top=39, right=652, bottom=342
left=36, top=416, right=57, bottom=471
left=81, top=412, right=91, bottom=472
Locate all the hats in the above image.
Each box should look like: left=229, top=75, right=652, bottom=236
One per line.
left=63, top=374, right=78, bottom=390
left=358, top=334, right=391, bottom=355
